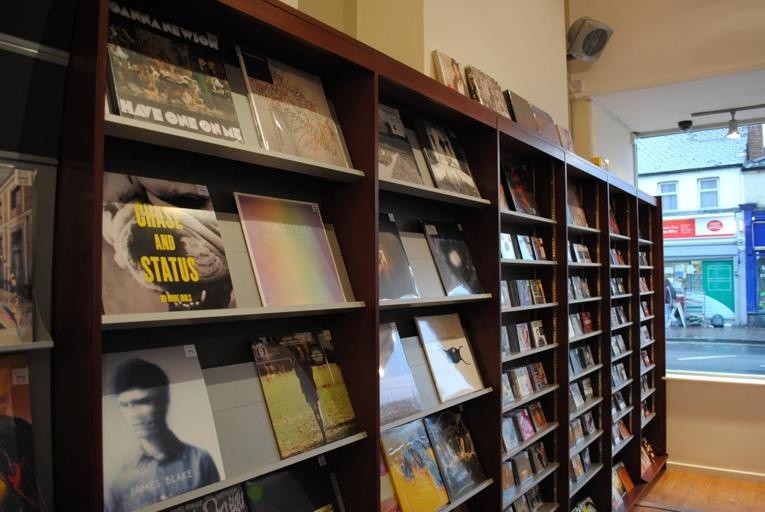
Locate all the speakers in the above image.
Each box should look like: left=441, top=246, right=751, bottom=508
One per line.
left=564, top=14, right=613, bottom=67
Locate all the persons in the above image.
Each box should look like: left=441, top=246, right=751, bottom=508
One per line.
left=106, top=357, right=221, bottom=512
left=290, top=345, right=328, bottom=444
left=664, top=277, right=681, bottom=329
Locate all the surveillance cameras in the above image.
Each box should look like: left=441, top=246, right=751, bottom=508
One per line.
left=678, top=119, right=692, bottom=133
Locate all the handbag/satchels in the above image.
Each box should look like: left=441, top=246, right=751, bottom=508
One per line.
left=666, top=286, right=676, bottom=309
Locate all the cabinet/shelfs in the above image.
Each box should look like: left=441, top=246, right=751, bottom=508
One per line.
left=1, top=1, right=669, bottom=512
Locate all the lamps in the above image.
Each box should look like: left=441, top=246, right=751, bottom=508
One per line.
left=724, top=112, right=742, bottom=137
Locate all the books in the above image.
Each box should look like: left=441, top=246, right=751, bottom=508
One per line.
left=569, top=410, right=596, bottom=453
left=567, top=239, right=593, bottom=263
left=570, top=446, right=592, bottom=483
left=612, top=419, right=630, bottom=445
left=568, top=345, right=596, bottom=379
left=500, top=231, right=548, bottom=261
left=610, top=248, right=625, bottom=265
left=378, top=312, right=485, bottom=425
left=232, top=43, right=357, bottom=170
left=503, top=161, right=543, bottom=217
left=611, top=363, right=628, bottom=387
left=641, top=437, right=657, bottom=478
left=243, top=450, right=354, bottom=511
left=500, top=441, right=549, bottom=494
left=0, top=31, right=73, bottom=68
left=500, top=400, right=548, bottom=454
left=610, top=334, right=626, bottom=358
left=379, top=404, right=493, bottom=512
left=101, top=169, right=238, bottom=317
left=104, top=0, right=245, bottom=144
left=501, top=360, right=549, bottom=406
left=500, top=278, right=548, bottom=308
left=566, top=179, right=601, bottom=230
left=568, top=376, right=594, bottom=414
left=233, top=191, right=348, bottom=309
left=0, top=159, right=59, bottom=347
left=501, top=318, right=548, bottom=358
left=503, top=88, right=575, bottom=153
left=611, top=391, right=628, bottom=417
left=466, top=64, right=512, bottom=120
left=610, top=305, right=628, bottom=327
left=103, top=344, right=226, bottom=510
left=0, top=351, right=41, bottom=512
left=638, top=251, right=652, bottom=347
left=377, top=212, right=486, bottom=304
left=377, top=101, right=483, bottom=200
left=612, top=461, right=634, bottom=509
left=503, top=485, right=545, bottom=512
left=432, top=49, right=471, bottom=98
left=250, top=328, right=360, bottom=460
left=639, top=349, right=652, bottom=421
left=568, top=310, right=593, bottom=339
left=610, top=277, right=626, bottom=296
left=568, top=275, right=592, bottom=302
